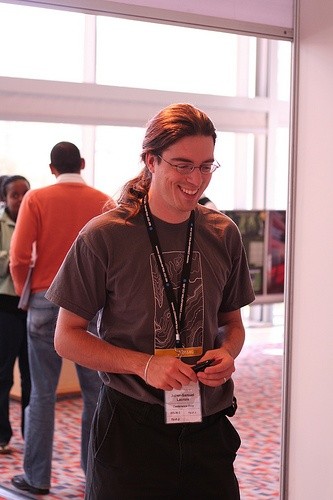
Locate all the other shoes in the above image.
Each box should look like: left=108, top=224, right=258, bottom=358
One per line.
left=0, top=444, right=11, bottom=454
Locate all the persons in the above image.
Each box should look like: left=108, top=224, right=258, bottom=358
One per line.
left=10, top=142, right=119, bottom=495
left=44, top=105, right=255, bottom=500
left=0, top=175, right=32, bottom=453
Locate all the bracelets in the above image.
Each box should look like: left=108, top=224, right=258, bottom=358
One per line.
left=145, top=355, right=153, bottom=383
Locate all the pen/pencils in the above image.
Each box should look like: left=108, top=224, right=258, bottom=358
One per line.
left=192, top=358, right=215, bottom=373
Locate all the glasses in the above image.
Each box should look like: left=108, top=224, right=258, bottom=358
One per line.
left=155, top=155, right=221, bottom=174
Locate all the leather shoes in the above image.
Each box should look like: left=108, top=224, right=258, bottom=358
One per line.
left=10, top=474, right=49, bottom=495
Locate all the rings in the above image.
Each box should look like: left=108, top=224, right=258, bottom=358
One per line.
left=224, top=377, right=227, bottom=383
